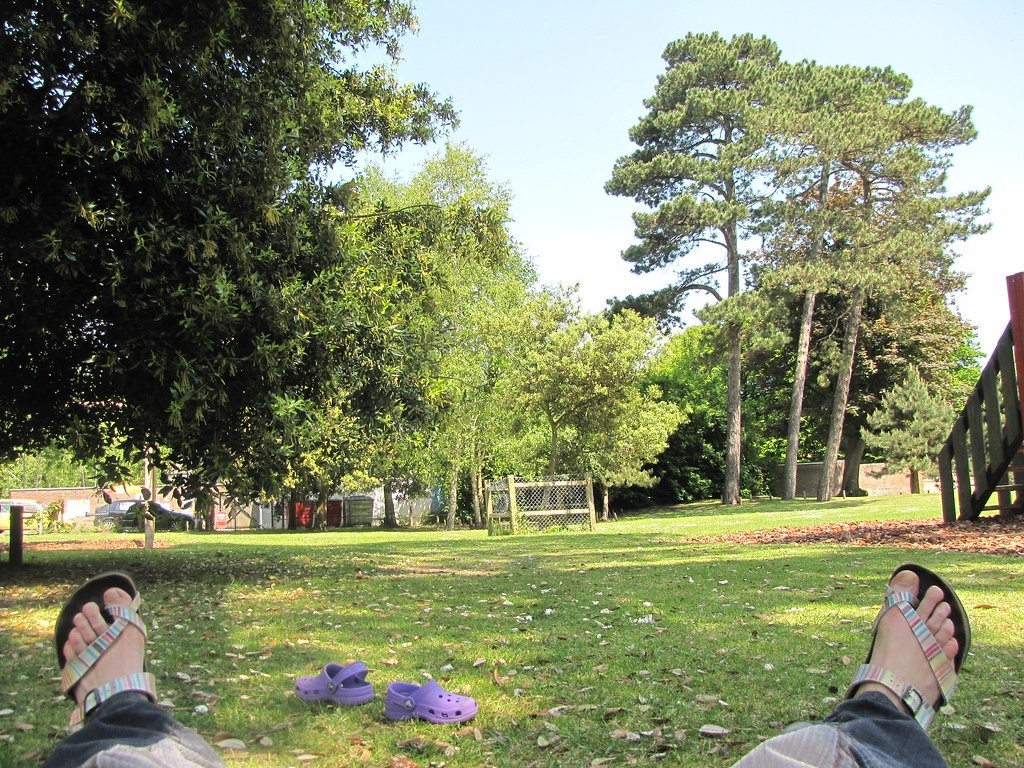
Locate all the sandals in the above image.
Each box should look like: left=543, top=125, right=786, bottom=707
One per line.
left=295, top=661, right=373, bottom=706
left=386, top=678, right=477, bottom=723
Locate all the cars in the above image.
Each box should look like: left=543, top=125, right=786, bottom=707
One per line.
left=0, top=499, right=51, bottom=532
left=93, top=499, right=195, bottom=532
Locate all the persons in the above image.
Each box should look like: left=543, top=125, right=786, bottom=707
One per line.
left=41, top=564, right=972, bottom=768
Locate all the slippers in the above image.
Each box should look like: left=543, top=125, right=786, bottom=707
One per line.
left=845, top=563, right=971, bottom=732
left=55, top=573, right=157, bottom=727
